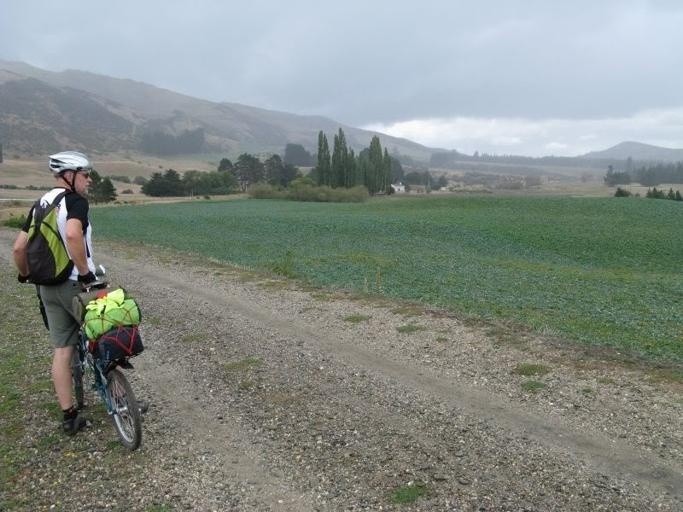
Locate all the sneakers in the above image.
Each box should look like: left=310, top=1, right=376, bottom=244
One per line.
left=63, top=414, right=85, bottom=436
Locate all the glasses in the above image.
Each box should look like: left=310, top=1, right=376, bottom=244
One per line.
left=76, top=172, right=90, bottom=179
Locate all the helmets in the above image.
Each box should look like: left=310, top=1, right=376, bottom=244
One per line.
left=47, top=150, right=92, bottom=175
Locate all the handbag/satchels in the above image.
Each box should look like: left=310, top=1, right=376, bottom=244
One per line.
left=72, top=285, right=144, bottom=362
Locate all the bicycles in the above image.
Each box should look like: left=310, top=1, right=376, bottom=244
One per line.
left=12, top=268, right=147, bottom=452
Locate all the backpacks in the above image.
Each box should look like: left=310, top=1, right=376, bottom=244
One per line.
left=25, top=190, right=74, bottom=287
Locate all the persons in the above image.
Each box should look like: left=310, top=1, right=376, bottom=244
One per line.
left=10, top=150, right=149, bottom=437
